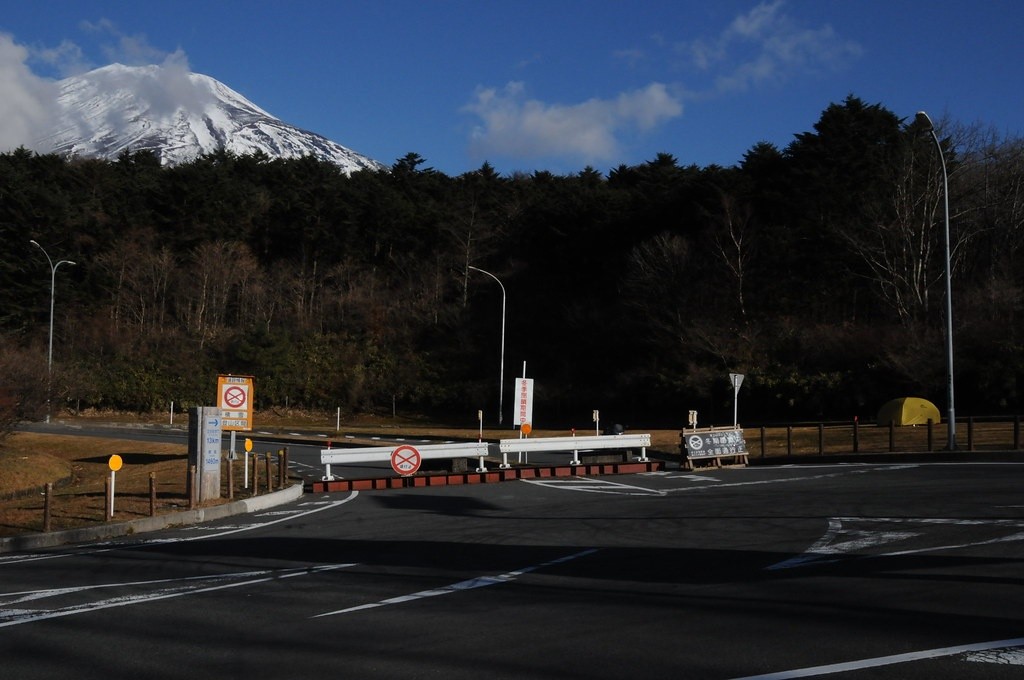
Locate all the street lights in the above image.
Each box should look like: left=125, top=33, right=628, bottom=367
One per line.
left=916, top=110, right=957, bottom=449
left=29, top=238, right=77, bottom=424
left=466, top=264, right=505, bottom=425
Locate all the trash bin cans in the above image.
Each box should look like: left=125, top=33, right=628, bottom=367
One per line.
left=603, top=424, right=624, bottom=451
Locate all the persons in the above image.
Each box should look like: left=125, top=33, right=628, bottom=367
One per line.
left=605, top=419, right=624, bottom=434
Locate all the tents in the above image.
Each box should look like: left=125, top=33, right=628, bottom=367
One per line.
left=878, top=397, right=941, bottom=426
